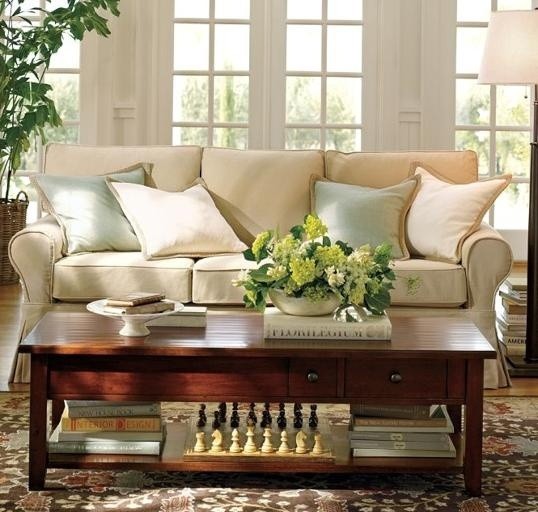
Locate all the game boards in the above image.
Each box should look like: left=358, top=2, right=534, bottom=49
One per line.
left=183, top=416, right=335, bottom=464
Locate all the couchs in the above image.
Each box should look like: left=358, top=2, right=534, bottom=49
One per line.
left=9, top=141, right=514, bottom=390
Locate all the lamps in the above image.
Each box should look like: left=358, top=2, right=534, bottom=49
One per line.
left=476, top=8, right=537, bottom=369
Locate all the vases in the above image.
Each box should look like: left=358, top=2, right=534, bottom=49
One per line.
left=266, top=286, right=340, bottom=316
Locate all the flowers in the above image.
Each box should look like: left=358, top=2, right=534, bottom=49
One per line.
left=230, top=211, right=422, bottom=324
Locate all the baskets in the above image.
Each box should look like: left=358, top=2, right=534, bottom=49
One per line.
left=0, top=190, right=29, bottom=286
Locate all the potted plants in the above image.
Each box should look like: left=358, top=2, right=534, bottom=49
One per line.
left=0, top=1, right=123, bottom=289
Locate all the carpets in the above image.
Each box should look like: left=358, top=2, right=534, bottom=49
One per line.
left=0, top=390, right=537, bottom=512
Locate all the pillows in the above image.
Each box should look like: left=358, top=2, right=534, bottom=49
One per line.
left=28, top=162, right=250, bottom=260
left=300, top=163, right=515, bottom=266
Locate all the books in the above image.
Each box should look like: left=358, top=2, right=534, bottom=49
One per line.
left=496, top=278, right=528, bottom=356
left=48, top=400, right=166, bottom=455
left=145, top=307, right=207, bottom=328
left=104, top=293, right=174, bottom=315
left=350, top=404, right=456, bottom=457
